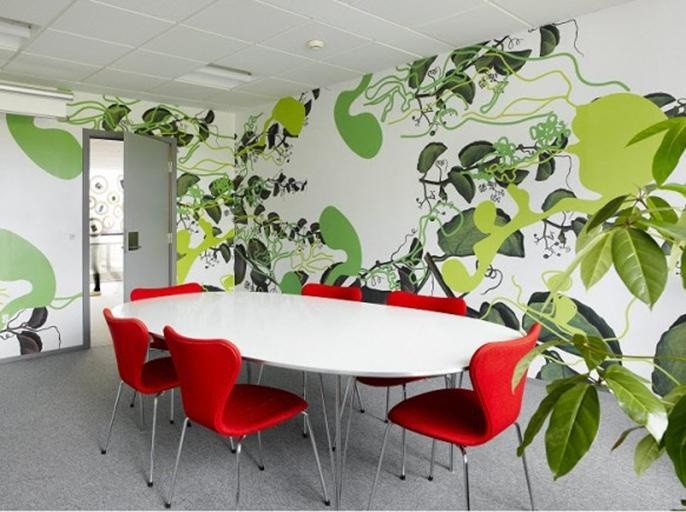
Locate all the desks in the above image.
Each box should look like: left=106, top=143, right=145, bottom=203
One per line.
left=112, top=291, right=525, bottom=508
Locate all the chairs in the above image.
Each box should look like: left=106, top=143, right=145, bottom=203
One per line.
left=255, top=283, right=366, bottom=438
left=337, top=293, right=467, bottom=480
left=102, top=307, right=238, bottom=487
left=162, top=326, right=330, bottom=509
left=364, top=323, right=541, bottom=510
left=126, top=283, right=204, bottom=425
